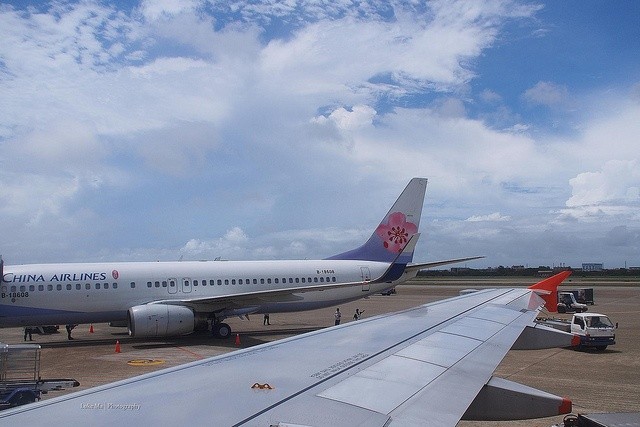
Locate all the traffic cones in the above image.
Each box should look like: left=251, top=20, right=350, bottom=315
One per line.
left=114, top=339, right=121, bottom=352
left=90, top=323, right=93, bottom=333
left=235, top=333, right=240, bottom=344
left=0, top=269, right=581, bottom=427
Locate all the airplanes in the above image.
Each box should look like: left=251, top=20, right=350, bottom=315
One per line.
left=0, top=178, right=487, bottom=339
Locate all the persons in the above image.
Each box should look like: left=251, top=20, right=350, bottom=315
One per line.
left=263, top=313, right=271, bottom=325
left=24, top=327, right=32, bottom=341
left=334, top=308, right=341, bottom=326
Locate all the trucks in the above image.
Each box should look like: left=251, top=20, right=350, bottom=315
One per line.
left=552, top=411, right=640, bottom=426
left=558, top=288, right=595, bottom=313
left=536, top=313, right=618, bottom=351
